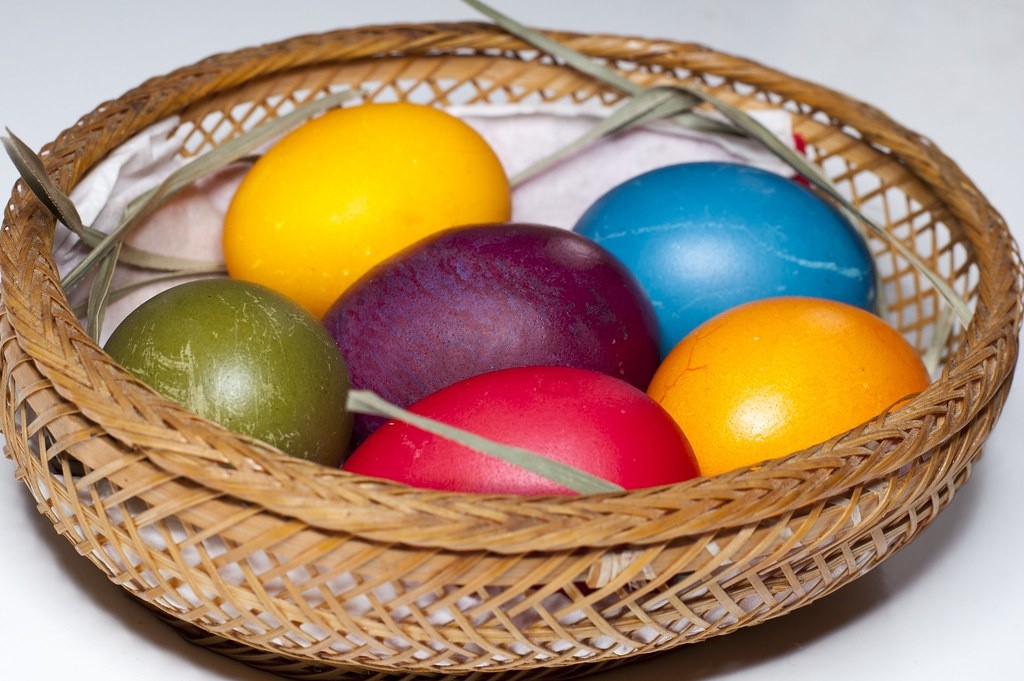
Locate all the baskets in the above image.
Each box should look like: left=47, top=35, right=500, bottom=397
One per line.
left=0, top=21, right=1024, bottom=677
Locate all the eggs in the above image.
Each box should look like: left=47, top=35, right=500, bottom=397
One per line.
left=100, top=97, right=931, bottom=502
